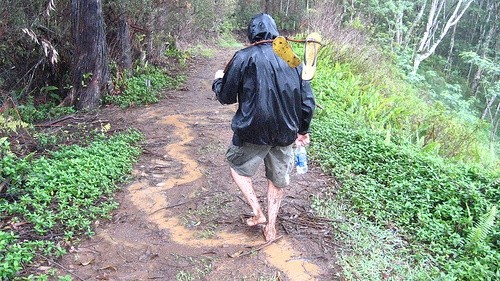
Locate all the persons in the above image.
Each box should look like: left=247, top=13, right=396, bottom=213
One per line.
left=211, top=14, right=315, bottom=242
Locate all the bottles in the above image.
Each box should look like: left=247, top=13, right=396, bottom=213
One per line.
left=294, top=145, right=308, bottom=175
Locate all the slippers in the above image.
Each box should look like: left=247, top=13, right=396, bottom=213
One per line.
left=301, top=33, right=322, bottom=81
left=272, top=37, right=301, bottom=67
